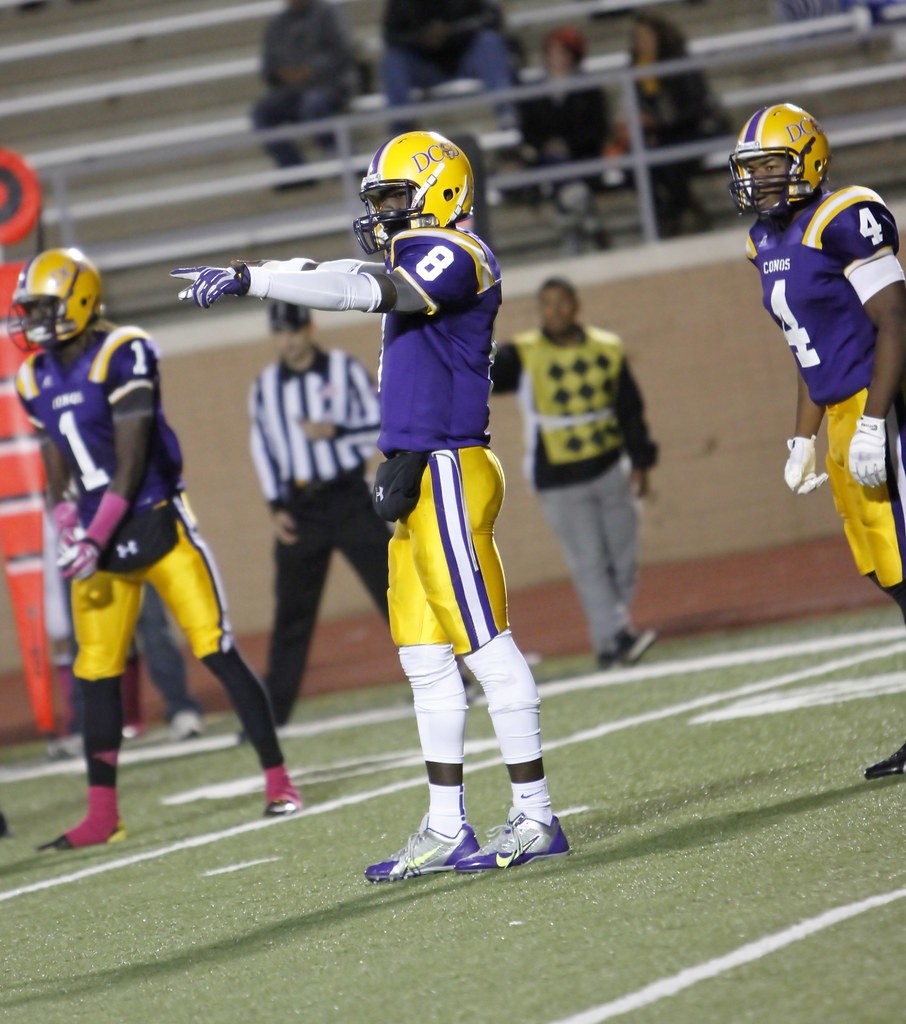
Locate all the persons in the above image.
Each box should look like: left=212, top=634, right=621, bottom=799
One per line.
left=251, top=0, right=906, bottom=258
left=729, top=103, right=906, bottom=780
left=488, top=278, right=658, bottom=671
left=168, top=129, right=570, bottom=882
left=7, top=246, right=300, bottom=854
left=42, top=296, right=471, bottom=758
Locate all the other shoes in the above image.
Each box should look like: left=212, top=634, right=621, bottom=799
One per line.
left=45, top=734, right=85, bottom=760
left=613, top=626, right=657, bottom=667
left=262, top=789, right=303, bottom=818
left=35, top=817, right=127, bottom=852
left=169, top=708, right=202, bottom=739
left=596, top=650, right=617, bottom=672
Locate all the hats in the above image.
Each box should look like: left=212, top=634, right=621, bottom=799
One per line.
left=267, top=298, right=312, bottom=331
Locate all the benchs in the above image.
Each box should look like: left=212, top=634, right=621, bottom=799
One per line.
left=0, top=1, right=906, bottom=315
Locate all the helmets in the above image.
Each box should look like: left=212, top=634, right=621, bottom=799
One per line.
left=728, top=103, right=832, bottom=233
left=7, top=247, right=104, bottom=352
left=353, top=131, right=475, bottom=255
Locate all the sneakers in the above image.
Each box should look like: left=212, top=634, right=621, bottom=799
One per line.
left=455, top=806, right=572, bottom=874
left=865, top=743, right=906, bottom=780
left=364, top=812, right=483, bottom=883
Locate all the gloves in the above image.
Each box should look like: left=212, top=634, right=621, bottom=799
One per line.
left=848, top=415, right=888, bottom=489
left=169, top=260, right=244, bottom=309
left=784, top=434, right=829, bottom=497
left=52, top=501, right=87, bottom=555
left=56, top=490, right=131, bottom=582
